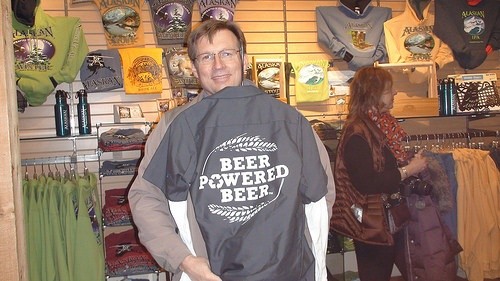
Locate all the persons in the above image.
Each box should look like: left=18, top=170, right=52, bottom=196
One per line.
left=126, top=18, right=338, bottom=281
left=330, top=66, right=431, bottom=280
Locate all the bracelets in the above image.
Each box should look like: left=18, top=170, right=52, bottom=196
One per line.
left=401, top=166, right=411, bottom=178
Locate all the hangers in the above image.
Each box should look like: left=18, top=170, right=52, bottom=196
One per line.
left=25, top=153, right=90, bottom=181
left=404, top=130, right=500, bottom=153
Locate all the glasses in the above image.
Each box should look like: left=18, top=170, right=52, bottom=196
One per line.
left=193, top=49, right=241, bottom=64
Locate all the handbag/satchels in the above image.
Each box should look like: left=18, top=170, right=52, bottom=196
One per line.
left=383, top=197, right=410, bottom=235
